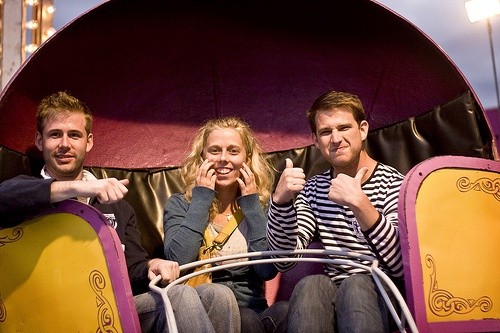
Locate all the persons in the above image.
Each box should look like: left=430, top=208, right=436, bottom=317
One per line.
left=163, top=116, right=290, bottom=333
left=266, top=88, right=407, bottom=333
left=0, top=91, right=241, bottom=333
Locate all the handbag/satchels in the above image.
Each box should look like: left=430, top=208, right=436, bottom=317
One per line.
left=181, top=246, right=213, bottom=286
